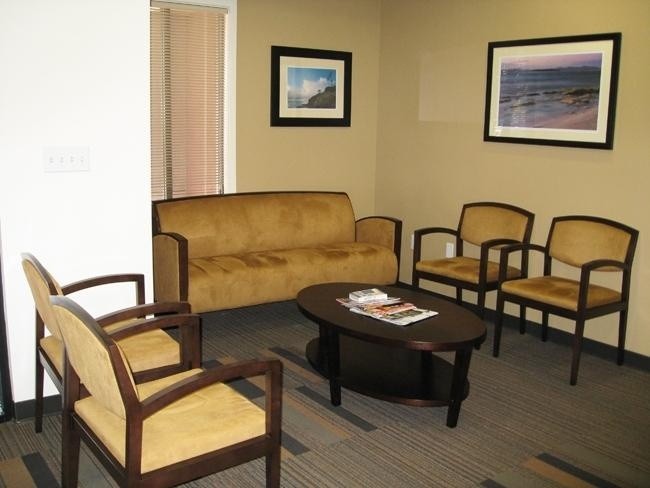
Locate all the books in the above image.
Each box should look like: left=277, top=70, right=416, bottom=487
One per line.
left=336, top=287, right=439, bottom=326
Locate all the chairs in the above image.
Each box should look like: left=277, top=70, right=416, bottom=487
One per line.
left=492, top=214, right=639, bottom=386
left=20, top=252, right=191, bottom=433
left=49, top=297, right=283, bottom=488
left=411, top=200, right=535, bottom=350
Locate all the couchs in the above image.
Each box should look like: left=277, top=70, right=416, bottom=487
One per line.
left=152, top=191, right=403, bottom=330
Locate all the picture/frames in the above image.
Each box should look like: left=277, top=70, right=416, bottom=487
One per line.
left=270, top=45, right=352, bottom=127
left=483, top=32, right=623, bottom=150
